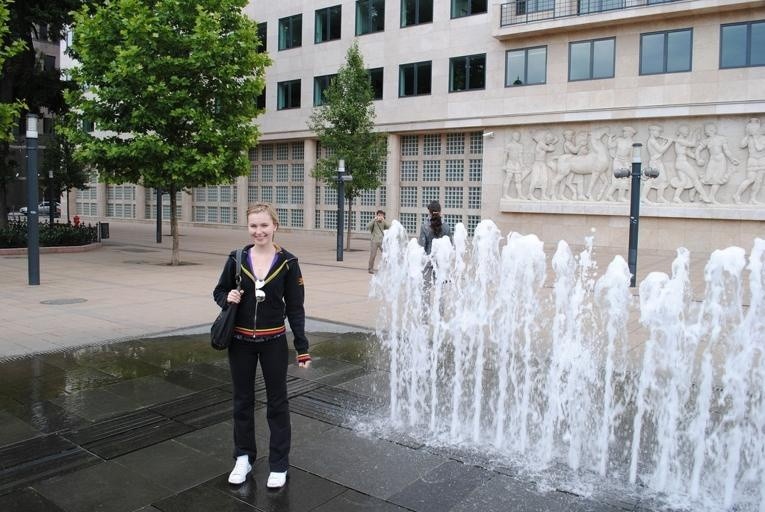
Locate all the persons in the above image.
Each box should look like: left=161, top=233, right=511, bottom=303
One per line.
left=419, top=199, right=452, bottom=290
left=366, top=209, right=390, bottom=274
left=500, top=117, right=764, bottom=206
left=213, top=203, right=311, bottom=490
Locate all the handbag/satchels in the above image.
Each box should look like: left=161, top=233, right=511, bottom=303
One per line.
left=210, top=249, right=244, bottom=351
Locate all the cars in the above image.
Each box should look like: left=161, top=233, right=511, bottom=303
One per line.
left=18, top=201, right=61, bottom=215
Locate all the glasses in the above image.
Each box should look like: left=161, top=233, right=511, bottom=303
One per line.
left=254, top=279, right=266, bottom=303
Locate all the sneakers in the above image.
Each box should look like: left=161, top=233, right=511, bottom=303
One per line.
left=267, top=470, right=289, bottom=488
left=368, top=267, right=372, bottom=274
left=229, top=455, right=253, bottom=484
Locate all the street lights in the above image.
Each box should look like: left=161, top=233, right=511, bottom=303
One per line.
left=613, top=141, right=659, bottom=288
left=25, top=114, right=41, bottom=286
left=331, top=159, right=352, bottom=262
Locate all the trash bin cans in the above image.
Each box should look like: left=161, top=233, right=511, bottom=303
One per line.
left=96, top=222, right=109, bottom=241
